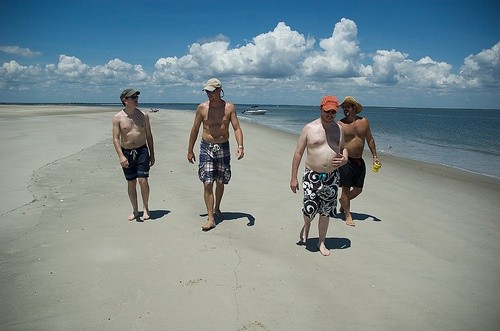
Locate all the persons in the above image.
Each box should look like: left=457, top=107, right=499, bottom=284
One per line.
left=290, top=96, right=348, bottom=256
left=336, top=96, right=382, bottom=226
left=113, top=88, right=155, bottom=221
left=187, top=78, right=244, bottom=229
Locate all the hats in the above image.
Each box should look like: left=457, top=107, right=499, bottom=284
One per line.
left=320, top=95, right=339, bottom=112
left=202, top=78, right=222, bottom=91
left=119, top=88, right=140, bottom=100
left=338, top=95, right=363, bottom=113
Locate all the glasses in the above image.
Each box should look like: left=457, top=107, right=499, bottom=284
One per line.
left=125, top=95, right=138, bottom=99
left=342, top=104, right=354, bottom=108
left=321, top=110, right=337, bottom=114
left=205, top=88, right=217, bottom=93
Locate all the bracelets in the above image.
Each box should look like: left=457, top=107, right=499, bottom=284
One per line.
left=238, top=146, right=244, bottom=149
left=373, top=156, right=379, bottom=158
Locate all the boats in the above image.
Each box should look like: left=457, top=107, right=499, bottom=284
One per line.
left=241, top=104, right=268, bottom=114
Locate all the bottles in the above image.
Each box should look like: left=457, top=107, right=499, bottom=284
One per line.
left=371, top=161, right=381, bottom=173
